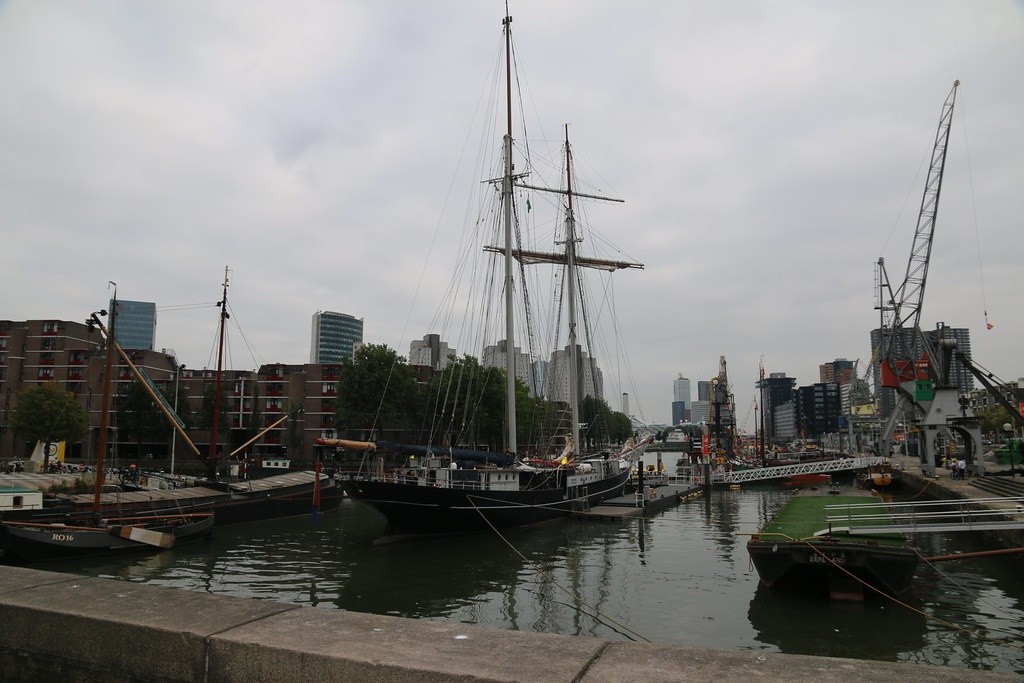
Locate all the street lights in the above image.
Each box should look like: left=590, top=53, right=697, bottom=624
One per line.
left=1003, top=423, right=1015, bottom=479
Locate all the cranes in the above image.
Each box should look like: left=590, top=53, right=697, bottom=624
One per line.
left=867, top=77, right=961, bottom=459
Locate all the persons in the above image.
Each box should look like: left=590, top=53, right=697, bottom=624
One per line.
left=956, top=458, right=966, bottom=480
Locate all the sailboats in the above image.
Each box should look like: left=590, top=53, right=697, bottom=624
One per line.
left=2, top=281, right=214, bottom=551
left=336, top=8, right=674, bottom=538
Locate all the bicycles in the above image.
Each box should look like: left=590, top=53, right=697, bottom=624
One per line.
left=950, top=468, right=960, bottom=480
